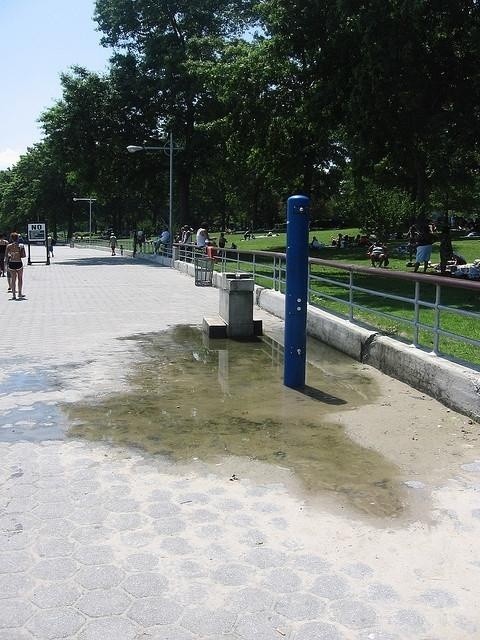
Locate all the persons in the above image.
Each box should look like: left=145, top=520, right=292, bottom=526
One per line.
left=240, top=230, right=252, bottom=241
left=231, top=242, right=237, bottom=258
left=0, top=232, right=27, bottom=300
left=109, top=233, right=117, bottom=255
left=139, top=233, right=146, bottom=247
left=217, top=232, right=226, bottom=257
left=48, top=235, right=54, bottom=258
left=405, top=224, right=420, bottom=263
left=132, top=230, right=140, bottom=259
left=150, top=228, right=170, bottom=256
left=432, top=215, right=480, bottom=240
left=180, top=226, right=186, bottom=241
left=367, top=238, right=388, bottom=269
left=181, top=224, right=192, bottom=263
left=197, top=221, right=209, bottom=245
left=438, top=225, right=454, bottom=275
left=310, top=233, right=370, bottom=250
left=452, top=253, right=468, bottom=266
left=413, top=232, right=439, bottom=272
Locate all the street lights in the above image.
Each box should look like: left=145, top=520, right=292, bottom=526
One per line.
left=73, top=196, right=97, bottom=244
left=126, top=130, right=184, bottom=246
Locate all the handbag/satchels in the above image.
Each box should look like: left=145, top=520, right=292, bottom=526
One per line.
left=20, top=245, right=26, bottom=258
